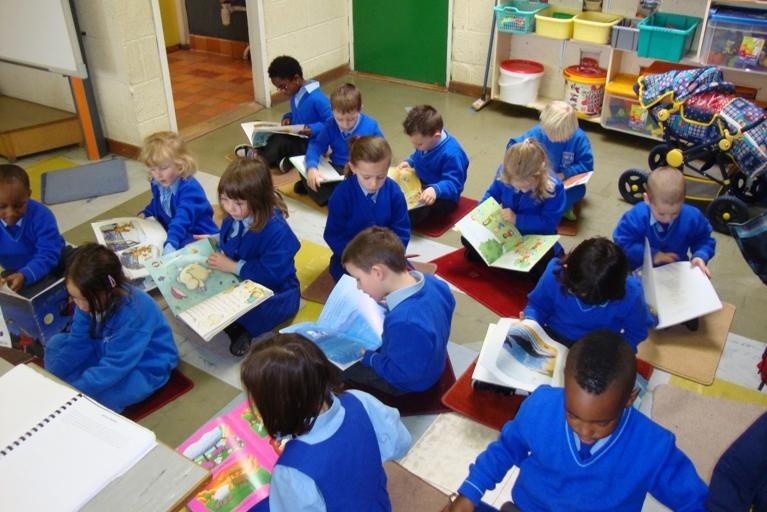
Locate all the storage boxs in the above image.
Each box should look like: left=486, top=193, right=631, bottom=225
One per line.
left=492, top=0, right=767, bottom=143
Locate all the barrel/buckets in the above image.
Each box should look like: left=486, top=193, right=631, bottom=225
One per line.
left=498, top=60, right=545, bottom=107
left=564, top=64, right=607, bottom=119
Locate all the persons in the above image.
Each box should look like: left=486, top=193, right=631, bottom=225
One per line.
left=294, top=82, right=387, bottom=207
left=449, top=330, right=712, bottom=511
left=613, top=166, right=719, bottom=334
left=133, top=129, right=219, bottom=251
left=396, top=104, right=470, bottom=225
left=505, top=99, right=594, bottom=220
left=234, top=54, right=331, bottom=174
left=473, top=236, right=654, bottom=398
left=43, top=242, right=180, bottom=413
left=331, top=226, right=454, bottom=399
left=242, top=332, right=415, bottom=511
left=461, top=137, right=566, bottom=284
left=323, top=133, right=416, bottom=283
left=191, top=158, right=301, bottom=357
left=1, top=164, right=77, bottom=298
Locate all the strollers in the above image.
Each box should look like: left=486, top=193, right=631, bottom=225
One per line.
left=617, top=58, right=767, bottom=233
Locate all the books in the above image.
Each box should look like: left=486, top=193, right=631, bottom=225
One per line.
left=88, top=214, right=177, bottom=292
left=452, top=195, right=560, bottom=275
left=475, top=316, right=650, bottom=410
left=385, top=166, right=428, bottom=212
left=144, top=237, right=275, bottom=341
left=563, top=170, right=593, bottom=190
left=288, top=154, right=347, bottom=186
left=278, top=273, right=386, bottom=371
left=241, top=121, right=308, bottom=149
left=633, top=237, right=722, bottom=329
left=1, top=279, right=76, bottom=358
left=175, top=396, right=293, bottom=512
left=0, top=361, right=159, bottom=512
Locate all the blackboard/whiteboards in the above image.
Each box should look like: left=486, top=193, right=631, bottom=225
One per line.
left=349, top=0, right=452, bottom=92
left=0, top=0, right=87, bottom=79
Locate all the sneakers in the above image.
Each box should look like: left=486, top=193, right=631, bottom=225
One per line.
left=234, top=144, right=263, bottom=161
left=225, top=322, right=250, bottom=355
left=294, top=180, right=307, bottom=194
left=471, top=379, right=515, bottom=395
left=275, top=155, right=293, bottom=173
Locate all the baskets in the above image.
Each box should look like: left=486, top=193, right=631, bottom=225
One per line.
left=494, top=0, right=550, bottom=34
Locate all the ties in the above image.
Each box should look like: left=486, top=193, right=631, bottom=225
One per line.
left=579, top=437, right=598, bottom=459
left=366, top=193, right=376, bottom=207
left=223, top=220, right=244, bottom=261
left=658, top=220, right=670, bottom=237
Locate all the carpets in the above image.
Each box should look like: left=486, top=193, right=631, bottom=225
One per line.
left=22, top=156, right=129, bottom=206
